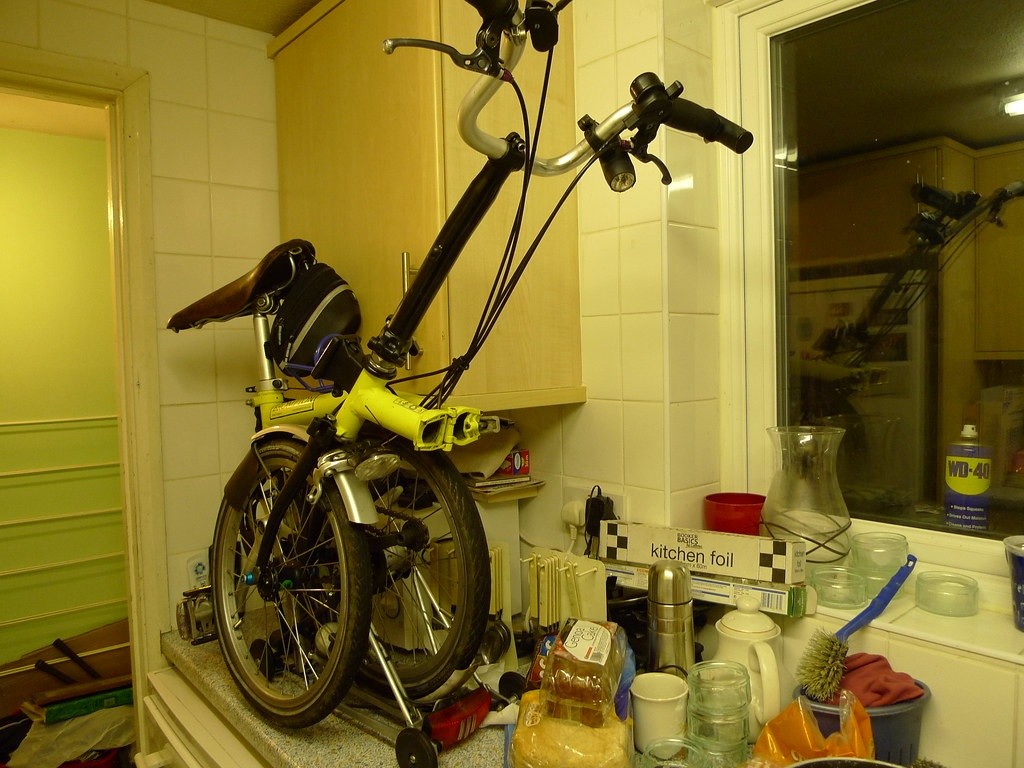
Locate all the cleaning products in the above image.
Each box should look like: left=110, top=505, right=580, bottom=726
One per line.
left=941, top=423, right=993, bottom=534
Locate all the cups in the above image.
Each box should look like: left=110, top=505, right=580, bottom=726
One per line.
left=703, top=490, right=767, bottom=535
left=1002, top=535, right=1024, bottom=632
left=630, top=672, right=689, bottom=757
left=915, top=570, right=979, bottom=618
left=687, top=658, right=752, bottom=768
left=639, top=737, right=706, bottom=768
left=809, top=565, right=868, bottom=609
left=709, top=595, right=799, bottom=744
left=851, top=531, right=910, bottom=600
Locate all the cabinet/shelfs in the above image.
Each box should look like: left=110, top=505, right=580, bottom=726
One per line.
left=266, top=0, right=586, bottom=415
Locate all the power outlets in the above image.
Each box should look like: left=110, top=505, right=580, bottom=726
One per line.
left=561, top=488, right=627, bottom=536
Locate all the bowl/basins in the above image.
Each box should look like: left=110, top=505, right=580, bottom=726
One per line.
left=783, top=756, right=903, bottom=768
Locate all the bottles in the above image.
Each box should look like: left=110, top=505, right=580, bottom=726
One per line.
left=760, top=424, right=852, bottom=576
left=942, top=423, right=994, bottom=533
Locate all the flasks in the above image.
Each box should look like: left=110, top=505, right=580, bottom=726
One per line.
left=645, top=558, right=696, bottom=677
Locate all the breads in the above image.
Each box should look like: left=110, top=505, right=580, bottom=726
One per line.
left=544, top=618, right=617, bottom=728
left=509, top=690, right=631, bottom=768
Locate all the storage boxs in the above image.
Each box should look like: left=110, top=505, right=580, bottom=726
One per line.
left=600, top=559, right=810, bottom=620
left=496, top=448, right=530, bottom=477
left=599, top=520, right=808, bottom=583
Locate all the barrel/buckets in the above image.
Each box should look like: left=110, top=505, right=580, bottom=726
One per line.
left=791, top=674, right=932, bottom=765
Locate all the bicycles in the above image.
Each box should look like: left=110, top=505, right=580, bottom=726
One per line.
left=163, top=2, right=754, bottom=768
left=786, top=175, right=1024, bottom=473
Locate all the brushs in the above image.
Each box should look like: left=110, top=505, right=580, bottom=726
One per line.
left=795, top=553, right=917, bottom=702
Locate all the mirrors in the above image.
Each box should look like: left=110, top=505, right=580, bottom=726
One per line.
left=767, top=0, right=1024, bottom=544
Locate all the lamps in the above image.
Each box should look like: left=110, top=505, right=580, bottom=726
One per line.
left=1004, top=98, right=1024, bottom=117
